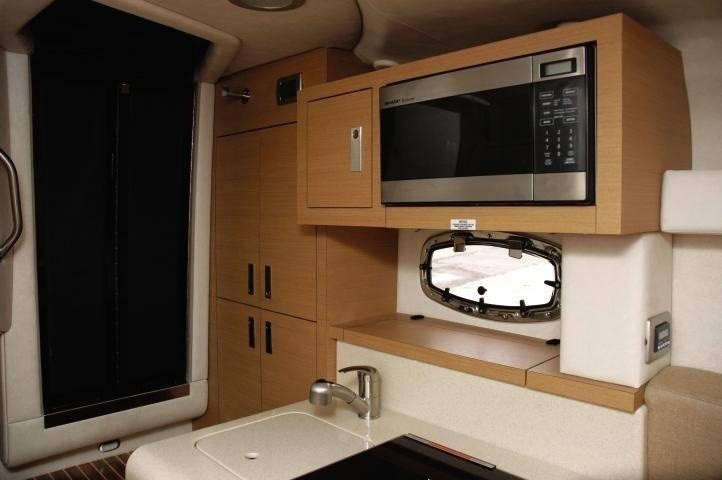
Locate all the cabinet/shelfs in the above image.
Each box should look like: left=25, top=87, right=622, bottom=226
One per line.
left=191, top=44, right=399, bottom=323
left=295, top=11, right=694, bottom=237
left=191, top=296, right=326, bottom=433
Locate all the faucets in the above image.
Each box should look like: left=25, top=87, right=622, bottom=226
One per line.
left=307, top=363, right=384, bottom=420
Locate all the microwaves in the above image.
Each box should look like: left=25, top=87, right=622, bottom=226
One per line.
left=378, top=44, right=596, bottom=206
